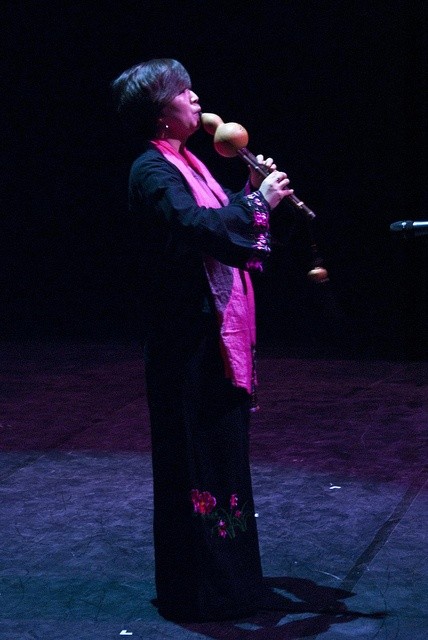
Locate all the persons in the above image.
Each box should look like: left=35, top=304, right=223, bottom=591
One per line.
left=113, top=59, right=292, bottom=622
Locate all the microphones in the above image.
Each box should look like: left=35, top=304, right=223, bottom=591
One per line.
left=389, top=220, right=428, bottom=239
